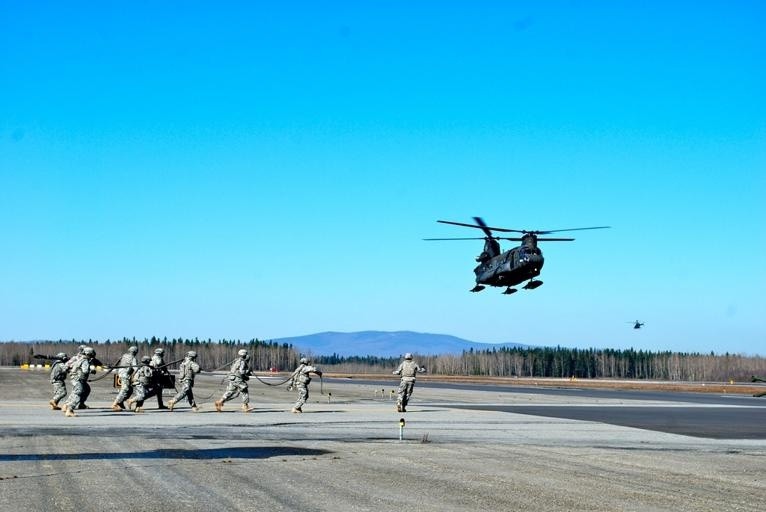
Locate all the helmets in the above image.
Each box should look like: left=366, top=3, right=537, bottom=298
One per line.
left=299, top=357, right=311, bottom=365
left=55, top=345, right=248, bottom=363
left=405, top=353, right=413, bottom=359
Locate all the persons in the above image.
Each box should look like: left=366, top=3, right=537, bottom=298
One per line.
left=142, top=348, right=171, bottom=410
left=125, top=355, right=154, bottom=413
left=166, top=349, right=204, bottom=412
left=48, top=352, right=70, bottom=410
left=61, top=346, right=97, bottom=418
left=64, top=343, right=103, bottom=410
left=289, top=356, right=323, bottom=413
left=112, top=345, right=140, bottom=412
left=214, top=348, right=255, bottom=413
left=391, top=352, right=427, bottom=412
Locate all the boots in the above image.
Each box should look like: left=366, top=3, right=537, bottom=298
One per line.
left=48, top=400, right=253, bottom=417
left=292, top=407, right=301, bottom=413
left=397, top=403, right=401, bottom=412
left=402, top=405, right=406, bottom=411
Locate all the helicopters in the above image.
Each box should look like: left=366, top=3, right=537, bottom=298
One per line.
left=425, top=216, right=610, bottom=295
left=626, top=319, right=648, bottom=332
